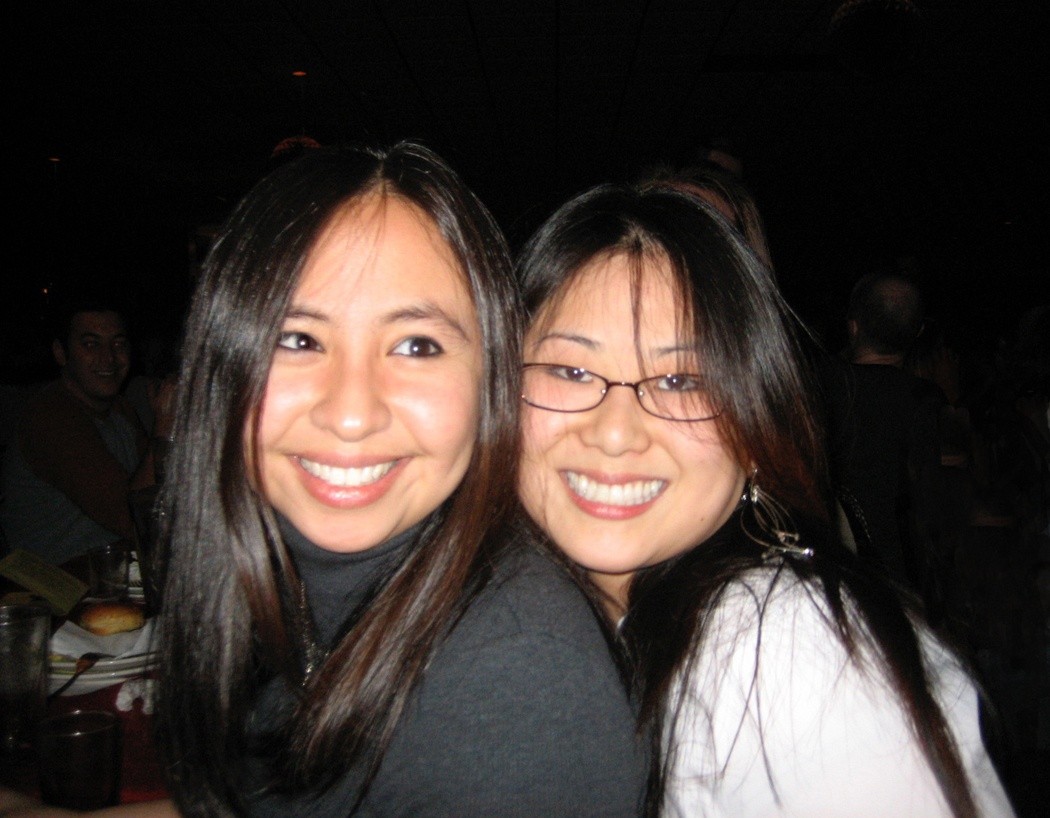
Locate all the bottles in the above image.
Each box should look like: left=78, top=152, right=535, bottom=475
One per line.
left=126, top=441, right=178, bottom=616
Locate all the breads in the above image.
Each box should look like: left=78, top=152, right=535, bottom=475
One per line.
left=78, top=601, right=144, bottom=636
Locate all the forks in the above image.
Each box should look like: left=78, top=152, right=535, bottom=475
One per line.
left=44, top=652, right=115, bottom=704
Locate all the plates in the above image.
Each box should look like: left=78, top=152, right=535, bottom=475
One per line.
left=105, top=580, right=145, bottom=605
left=46, top=616, right=166, bottom=678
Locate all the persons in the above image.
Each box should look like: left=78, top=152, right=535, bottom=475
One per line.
left=144, top=138, right=654, bottom=818
left=17, top=296, right=180, bottom=537
left=820, top=267, right=1050, bottom=633
left=511, top=159, right=1012, bottom=818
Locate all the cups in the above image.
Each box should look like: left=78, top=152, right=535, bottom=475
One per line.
left=88, top=544, right=131, bottom=601
left=34, top=710, right=122, bottom=812
left=0, top=605, right=51, bottom=764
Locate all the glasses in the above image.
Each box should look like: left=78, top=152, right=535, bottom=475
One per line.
left=515, top=362, right=731, bottom=421
